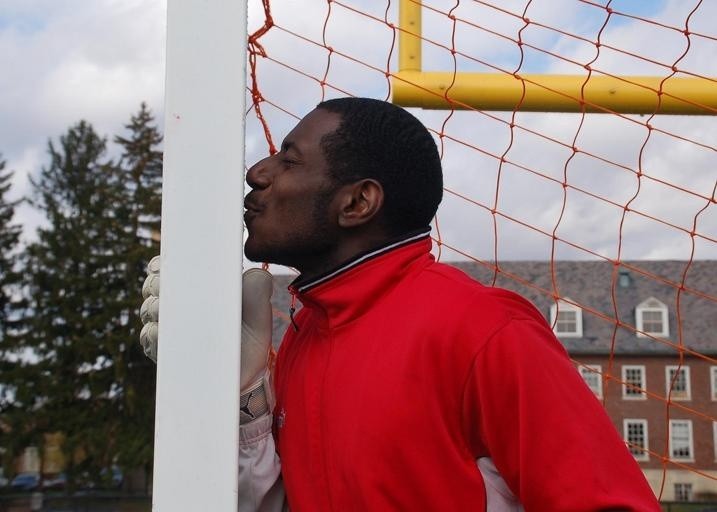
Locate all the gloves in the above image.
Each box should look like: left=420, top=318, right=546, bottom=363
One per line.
left=140, top=255, right=277, bottom=425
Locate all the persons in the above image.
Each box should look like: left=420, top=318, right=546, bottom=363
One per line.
left=139, top=97, right=662, bottom=512
